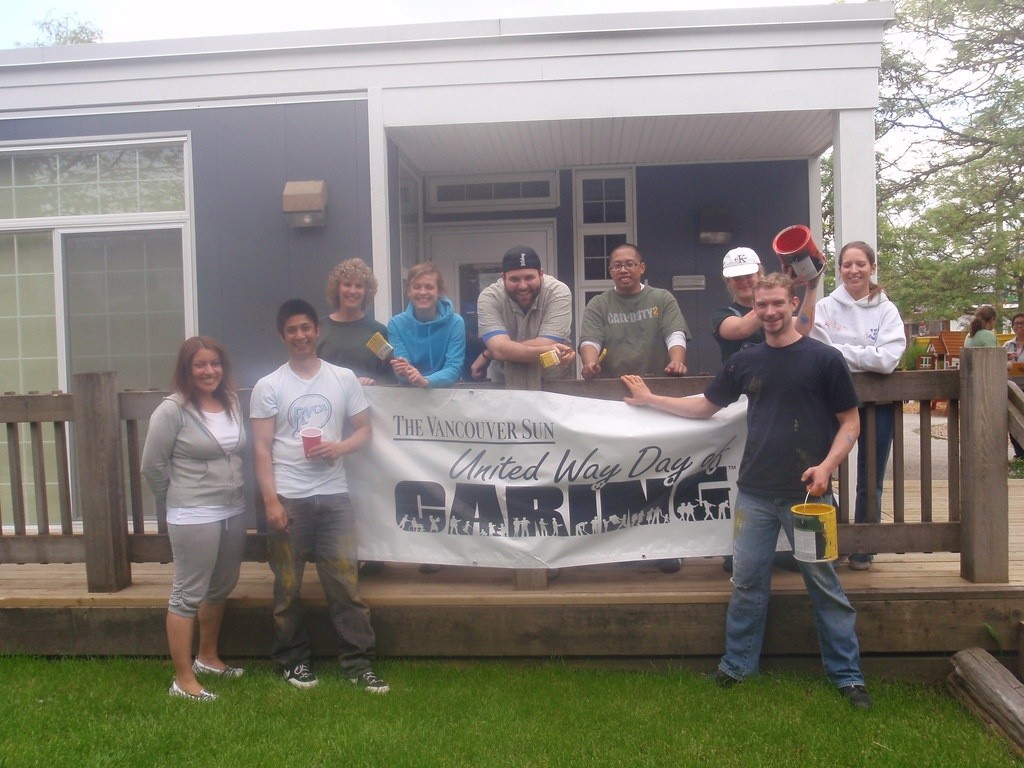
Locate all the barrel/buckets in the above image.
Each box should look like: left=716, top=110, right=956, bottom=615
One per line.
left=773, top=225, right=827, bottom=281
left=790, top=490, right=839, bottom=562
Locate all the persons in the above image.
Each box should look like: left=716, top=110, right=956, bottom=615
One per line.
left=1002, top=313, right=1024, bottom=460
left=709, top=245, right=826, bottom=573
left=138, top=337, right=249, bottom=701
left=248, top=299, right=391, bottom=695
left=314, top=256, right=387, bottom=575
left=621, top=271, right=871, bottom=715
left=385, top=261, right=466, bottom=572
left=963, top=305, right=1000, bottom=349
left=808, top=241, right=906, bottom=572
left=578, top=242, right=694, bottom=573
left=469, top=245, right=575, bottom=384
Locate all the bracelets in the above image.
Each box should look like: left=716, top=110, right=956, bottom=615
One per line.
left=482, top=351, right=491, bottom=362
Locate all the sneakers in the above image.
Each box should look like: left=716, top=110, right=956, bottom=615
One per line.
left=283, top=663, right=320, bottom=690
left=349, top=672, right=391, bottom=694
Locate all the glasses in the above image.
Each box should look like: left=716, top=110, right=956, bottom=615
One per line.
left=611, top=262, right=641, bottom=271
left=1013, top=322, right=1024, bottom=326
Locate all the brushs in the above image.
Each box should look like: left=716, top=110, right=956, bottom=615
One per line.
left=366, top=332, right=394, bottom=362
left=539, top=350, right=572, bottom=368
left=593, top=349, right=607, bottom=370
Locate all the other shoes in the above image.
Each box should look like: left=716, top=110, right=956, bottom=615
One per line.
left=834, top=554, right=872, bottom=570
left=838, top=686, right=873, bottom=711
left=713, top=668, right=742, bottom=689
left=168, top=681, right=218, bottom=702
left=192, top=655, right=245, bottom=678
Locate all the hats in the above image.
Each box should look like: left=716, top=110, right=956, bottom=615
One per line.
left=502, top=245, right=541, bottom=271
left=722, top=247, right=762, bottom=278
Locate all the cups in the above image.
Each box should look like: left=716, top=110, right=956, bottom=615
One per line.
left=300, top=427, right=323, bottom=459
